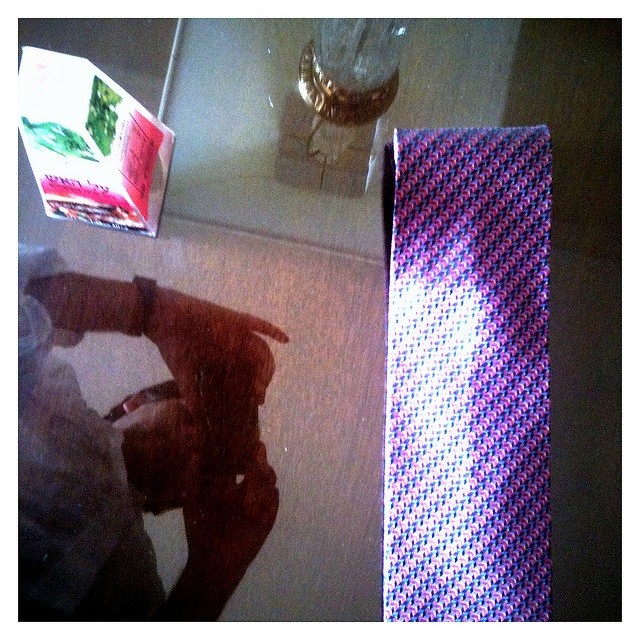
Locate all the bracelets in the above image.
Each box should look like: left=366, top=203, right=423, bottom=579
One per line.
left=133, top=276, right=156, bottom=335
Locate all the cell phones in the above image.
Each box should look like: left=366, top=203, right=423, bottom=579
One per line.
left=197, top=359, right=260, bottom=477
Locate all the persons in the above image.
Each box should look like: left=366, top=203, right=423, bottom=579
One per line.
left=18, top=247, right=289, bottom=622
